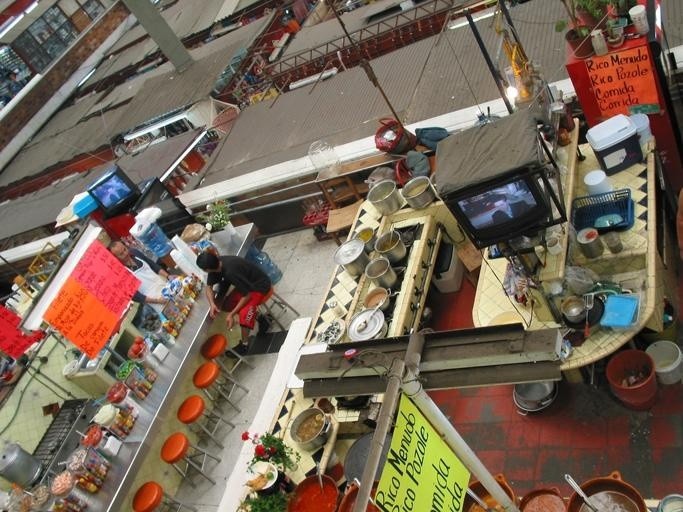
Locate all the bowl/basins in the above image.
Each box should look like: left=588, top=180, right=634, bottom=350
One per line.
left=106, top=383, right=126, bottom=404
left=29, top=485, right=50, bottom=510
left=657, top=493, right=683, bottom=512
left=78, top=424, right=102, bottom=449
left=92, top=402, right=115, bottom=427
left=127, top=342, right=147, bottom=362
left=50, top=470, right=74, bottom=497
left=61, top=360, right=78, bottom=376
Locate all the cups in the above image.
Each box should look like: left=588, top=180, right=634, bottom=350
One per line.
left=2, top=372, right=12, bottom=381
left=545, top=237, right=562, bottom=257
left=168, top=235, right=207, bottom=283
left=603, top=231, right=622, bottom=253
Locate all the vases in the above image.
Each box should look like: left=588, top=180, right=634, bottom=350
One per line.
left=278, top=454, right=298, bottom=472
left=223, top=221, right=236, bottom=236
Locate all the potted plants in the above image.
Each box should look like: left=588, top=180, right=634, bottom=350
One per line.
left=573, top=0, right=607, bottom=33
left=556, top=18, right=596, bottom=59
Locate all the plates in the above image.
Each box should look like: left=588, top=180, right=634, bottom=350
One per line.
left=5, top=366, right=22, bottom=384
left=249, top=461, right=278, bottom=489
left=320, top=319, right=343, bottom=345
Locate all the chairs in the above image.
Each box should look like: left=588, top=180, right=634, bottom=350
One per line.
left=317, top=175, right=364, bottom=247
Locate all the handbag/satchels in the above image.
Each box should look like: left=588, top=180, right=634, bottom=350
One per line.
left=374, top=118, right=417, bottom=152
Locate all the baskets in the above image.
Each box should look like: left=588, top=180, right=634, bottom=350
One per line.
left=573, top=188, right=631, bottom=231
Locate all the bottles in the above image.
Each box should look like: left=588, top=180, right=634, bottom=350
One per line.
left=557, top=129, right=569, bottom=146
left=164, top=164, right=191, bottom=200
left=160, top=272, right=201, bottom=337
left=52, top=496, right=88, bottom=512
left=663, top=313, right=672, bottom=323
left=328, top=301, right=344, bottom=318
left=127, top=366, right=156, bottom=400
left=105, top=403, right=138, bottom=439
left=71, top=452, right=111, bottom=494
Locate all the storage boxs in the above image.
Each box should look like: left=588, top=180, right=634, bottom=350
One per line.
left=431, top=243, right=464, bottom=294
left=586, top=114, right=643, bottom=176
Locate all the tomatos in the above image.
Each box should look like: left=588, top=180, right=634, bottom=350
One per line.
left=127, top=336, right=145, bottom=358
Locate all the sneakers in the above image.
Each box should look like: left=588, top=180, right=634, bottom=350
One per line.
left=226, top=341, right=249, bottom=358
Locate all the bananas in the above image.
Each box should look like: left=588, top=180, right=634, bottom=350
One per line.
left=245, top=475, right=267, bottom=492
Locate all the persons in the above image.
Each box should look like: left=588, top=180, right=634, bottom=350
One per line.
left=196, top=251, right=273, bottom=359
left=106, top=186, right=129, bottom=204
left=492, top=194, right=529, bottom=227
left=106, top=240, right=180, bottom=313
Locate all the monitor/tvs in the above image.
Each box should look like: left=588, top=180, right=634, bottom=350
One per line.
left=87, top=165, right=140, bottom=216
left=447, top=168, right=547, bottom=250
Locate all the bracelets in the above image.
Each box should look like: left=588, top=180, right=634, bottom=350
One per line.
left=166, top=274, right=171, bottom=280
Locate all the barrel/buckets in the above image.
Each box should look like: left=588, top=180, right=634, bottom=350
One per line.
left=642, top=303, right=678, bottom=345
left=583, top=169, right=617, bottom=204
left=249, top=247, right=282, bottom=284
left=629, top=113, right=653, bottom=144
left=646, top=340, right=683, bottom=386
left=309, top=140, right=341, bottom=178
left=605, top=349, right=659, bottom=410
left=135, top=223, right=174, bottom=258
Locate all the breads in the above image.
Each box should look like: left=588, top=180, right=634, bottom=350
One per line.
left=181, top=224, right=211, bottom=244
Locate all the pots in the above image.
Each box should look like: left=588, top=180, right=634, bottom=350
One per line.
left=509, top=382, right=558, bottom=418
left=285, top=475, right=342, bottom=511
left=0, top=443, right=43, bottom=491
left=347, top=308, right=388, bottom=340
left=460, top=467, right=646, bottom=512
left=333, top=227, right=406, bottom=289
left=288, top=409, right=332, bottom=450
left=400, top=176, right=433, bottom=211
left=559, top=297, right=585, bottom=326
left=366, top=179, right=402, bottom=217
left=358, top=287, right=392, bottom=311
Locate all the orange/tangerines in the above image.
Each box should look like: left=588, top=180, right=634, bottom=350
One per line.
left=267, top=473, right=274, bottom=479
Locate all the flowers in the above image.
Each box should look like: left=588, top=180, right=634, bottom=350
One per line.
left=195, top=191, right=232, bottom=233
left=242, top=431, right=301, bottom=473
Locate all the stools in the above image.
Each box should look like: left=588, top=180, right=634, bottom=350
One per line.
left=178, top=395, right=235, bottom=449
left=193, top=362, right=249, bottom=413
left=457, top=243, right=485, bottom=289
left=257, top=288, right=300, bottom=334
left=161, top=433, right=220, bottom=489
left=201, top=334, right=256, bottom=387
left=133, top=482, right=200, bottom=512
